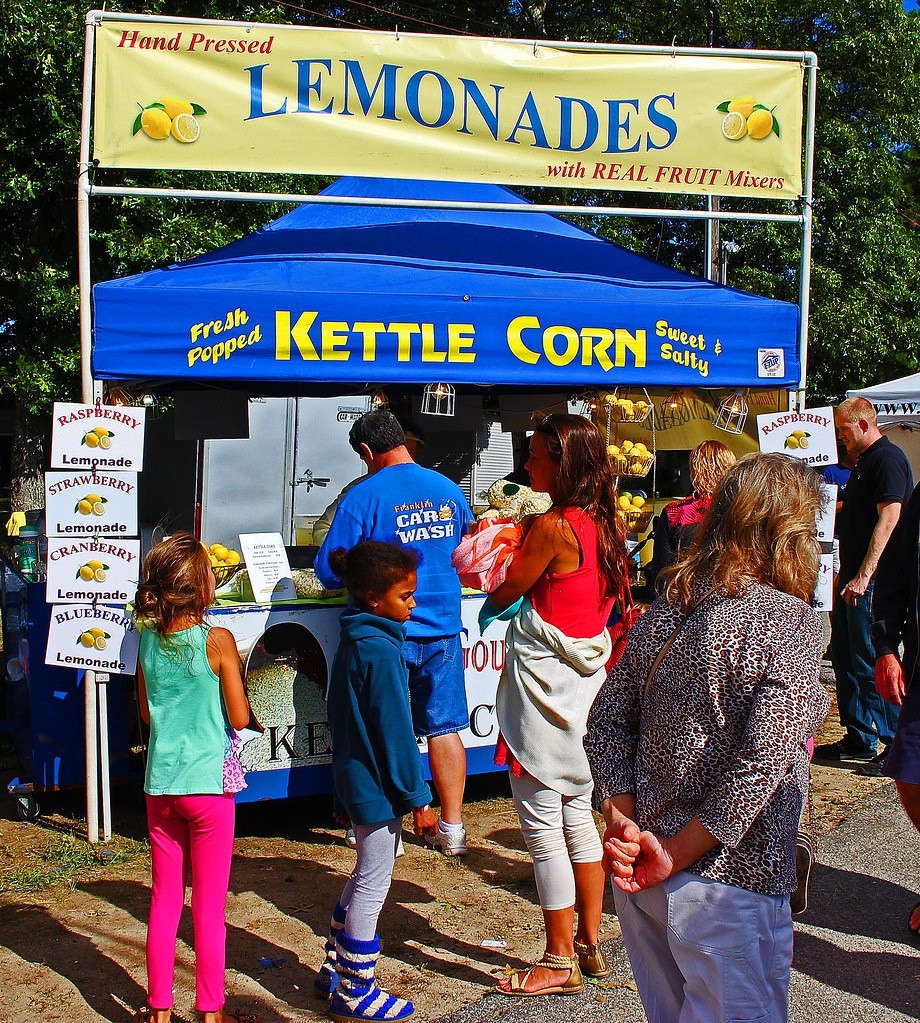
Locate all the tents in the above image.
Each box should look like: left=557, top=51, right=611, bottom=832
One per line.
left=846, top=371, right=919, bottom=490
left=90, top=175, right=802, bottom=839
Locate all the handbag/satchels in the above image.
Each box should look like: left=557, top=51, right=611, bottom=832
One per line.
left=602, top=517, right=642, bottom=678
left=790, top=831, right=814, bottom=915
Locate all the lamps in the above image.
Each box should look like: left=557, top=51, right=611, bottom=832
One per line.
left=660, top=389, right=691, bottom=423
left=367, top=385, right=390, bottom=414
left=579, top=388, right=604, bottom=418
left=710, top=389, right=749, bottom=435
left=420, top=382, right=456, bottom=417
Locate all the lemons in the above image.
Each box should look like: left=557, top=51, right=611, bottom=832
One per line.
left=722, top=96, right=773, bottom=140
left=787, top=429, right=808, bottom=449
left=80, top=627, right=107, bottom=650
left=618, top=492, right=652, bottom=532
left=200, top=542, right=240, bottom=583
left=603, top=395, right=648, bottom=420
left=85, top=426, right=112, bottom=449
left=136, top=96, right=200, bottom=142
left=77, top=494, right=105, bottom=515
left=606, top=439, right=654, bottom=475
left=79, top=560, right=107, bottom=582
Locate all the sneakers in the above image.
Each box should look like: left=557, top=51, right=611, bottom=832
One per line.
left=345, top=829, right=405, bottom=858
left=424, top=823, right=468, bottom=857
left=814, top=734, right=877, bottom=760
left=857, top=747, right=894, bottom=777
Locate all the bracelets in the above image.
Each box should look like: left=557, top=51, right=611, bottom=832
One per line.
left=413, top=804, right=429, bottom=815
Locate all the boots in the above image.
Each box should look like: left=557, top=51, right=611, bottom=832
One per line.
left=314, top=900, right=348, bottom=1000
left=330, top=927, right=415, bottom=1023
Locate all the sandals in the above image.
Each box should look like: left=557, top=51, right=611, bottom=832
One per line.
left=572, top=936, right=610, bottom=978
left=495, top=951, right=585, bottom=997
left=135, top=1007, right=172, bottom=1023
left=908, top=901, right=920, bottom=935
left=225, top=1006, right=259, bottom=1023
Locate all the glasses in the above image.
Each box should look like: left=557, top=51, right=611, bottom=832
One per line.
left=542, top=414, right=554, bottom=435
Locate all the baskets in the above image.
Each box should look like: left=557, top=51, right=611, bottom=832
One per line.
left=603, top=404, right=653, bottom=423
left=608, top=453, right=654, bottom=478
left=618, top=511, right=654, bottom=533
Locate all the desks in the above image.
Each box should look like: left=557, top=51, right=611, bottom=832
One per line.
left=204, top=587, right=518, bottom=806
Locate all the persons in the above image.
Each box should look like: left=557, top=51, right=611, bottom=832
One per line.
left=813, top=398, right=913, bottom=765
left=311, top=426, right=419, bottom=546
left=314, top=539, right=438, bottom=1023
left=580, top=450, right=827, bottom=1023
left=313, top=408, right=476, bottom=857
left=869, top=480, right=919, bottom=936
left=134, top=533, right=251, bottom=1023
left=641, top=439, right=736, bottom=592
left=487, top=413, right=627, bottom=996
